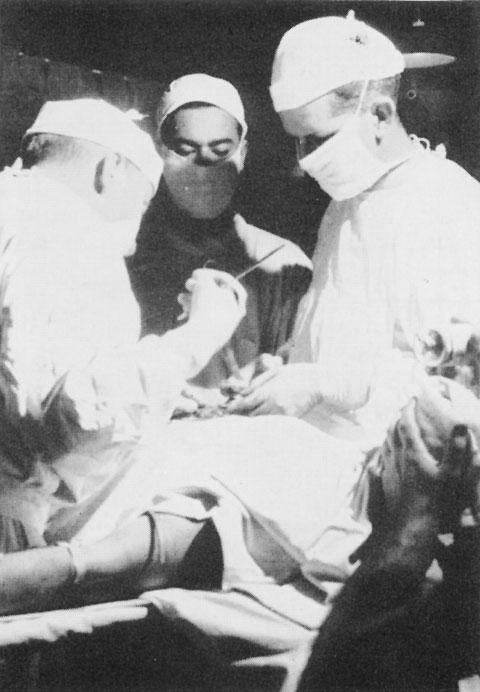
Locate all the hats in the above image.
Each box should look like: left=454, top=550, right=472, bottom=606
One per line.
left=158, top=74, right=247, bottom=141
left=270, top=17, right=456, bottom=114
left=23, top=98, right=164, bottom=198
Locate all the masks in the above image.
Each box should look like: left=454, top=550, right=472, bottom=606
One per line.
left=296, top=81, right=395, bottom=201
left=157, top=145, right=244, bottom=220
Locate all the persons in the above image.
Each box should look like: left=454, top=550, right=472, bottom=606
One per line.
left=0, top=9, right=480, bottom=692
